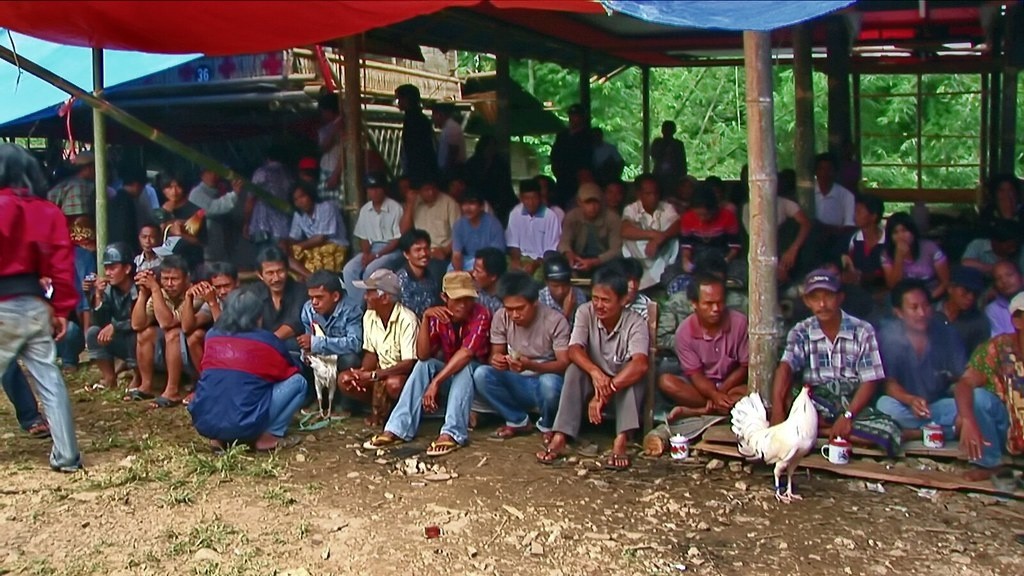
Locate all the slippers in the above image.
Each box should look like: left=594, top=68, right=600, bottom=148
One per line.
left=490, top=426, right=531, bottom=438
left=605, top=452, right=628, bottom=470
left=210, top=446, right=221, bottom=451
left=1017, top=476, right=1024, bottom=489
left=543, top=432, right=553, bottom=449
left=122, top=390, right=154, bottom=402
left=990, top=467, right=1017, bottom=491
left=26, top=419, right=50, bottom=438
left=537, top=449, right=567, bottom=463
left=427, top=441, right=462, bottom=455
left=146, top=397, right=178, bottom=408
left=363, top=435, right=403, bottom=449
left=255, top=435, right=301, bottom=453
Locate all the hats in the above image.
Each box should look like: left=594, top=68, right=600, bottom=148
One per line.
left=75, top=151, right=94, bottom=165
left=352, top=268, right=401, bottom=295
left=298, top=158, right=317, bottom=169
left=949, top=265, right=986, bottom=293
left=576, top=182, right=601, bottom=202
left=543, top=257, right=571, bottom=281
left=1008, top=292, right=1024, bottom=314
left=805, top=269, right=840, bottom=295
left=442, top=271, right=479, bottom=299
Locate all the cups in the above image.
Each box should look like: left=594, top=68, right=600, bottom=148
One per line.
left=923, top=422, right=944, bottom=448
left=821, top=436, right=850, bottom=464
left=669, top=433, right=688, bottom=460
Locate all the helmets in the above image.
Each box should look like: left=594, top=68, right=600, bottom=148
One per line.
left=363, top=173, right=385, bottom=187
left=100, top=242, right=135, bottom=264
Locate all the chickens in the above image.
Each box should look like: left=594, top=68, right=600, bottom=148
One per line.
left=300, top=321, right=337, bottom=419
left=160, top=208, right=206, bottom=237
left=730, top=384, right=818, bottom=505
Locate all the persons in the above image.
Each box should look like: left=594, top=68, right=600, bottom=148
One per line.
left=0, top=86, right=363, bottom=473
left=384, top=85, right=465, bottom=202
left=650, top=120, right=687, bottom=200
left=549, top=103, right=623, bottom=209
left=465, top=135, right=519, bottom=229
left=771, top=151, right=1024, bottom=477
left=343, top=165, right=749, bottom=471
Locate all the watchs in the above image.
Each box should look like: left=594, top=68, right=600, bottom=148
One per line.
left=841, top=409, right=854, bottom=421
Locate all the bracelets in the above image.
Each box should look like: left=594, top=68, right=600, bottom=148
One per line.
left=209, top=301, right=217, bottom=307
left=371, top=370, right=379, bottom=382
left=374, top=252, right=379, bottom=259
